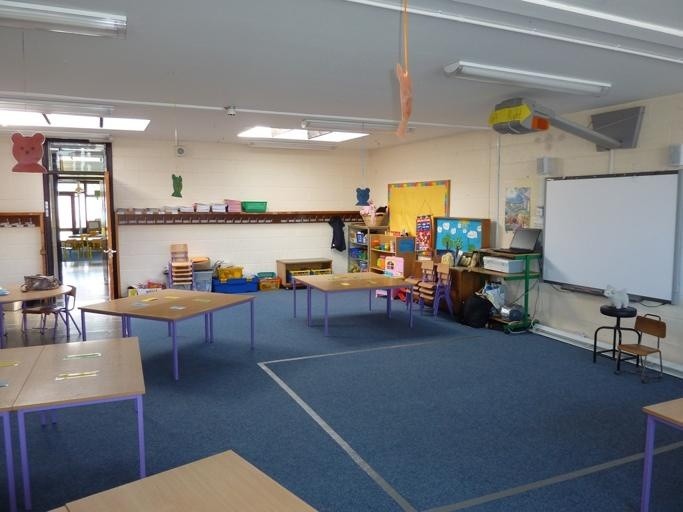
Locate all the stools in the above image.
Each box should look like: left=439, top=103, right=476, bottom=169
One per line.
left=593, top=304, right=642, bottom=370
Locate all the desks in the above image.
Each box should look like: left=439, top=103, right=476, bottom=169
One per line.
left=292, top=271, right=414, bottom=337
left=470, top=247, right=541, bottom=335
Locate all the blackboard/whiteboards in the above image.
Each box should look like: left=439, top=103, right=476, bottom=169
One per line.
left=541, top=170, right=681, bottom=305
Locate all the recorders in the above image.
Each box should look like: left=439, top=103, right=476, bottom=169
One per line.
left=500, top=302, right=524, bottom=322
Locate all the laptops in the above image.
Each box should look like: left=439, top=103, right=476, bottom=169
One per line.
left=494, top=226, right=542, bottom=255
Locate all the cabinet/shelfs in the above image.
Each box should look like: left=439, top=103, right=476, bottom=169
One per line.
left=275, top=257, right=332, bottom=289
left=343, top=222, right=415, bottom=274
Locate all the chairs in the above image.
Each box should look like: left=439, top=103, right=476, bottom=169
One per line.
left=168, top=243, right=195, bottom=277
left=617, top=312, right=667, bottom=383
left=402, top=259, right=455, bottom=320
